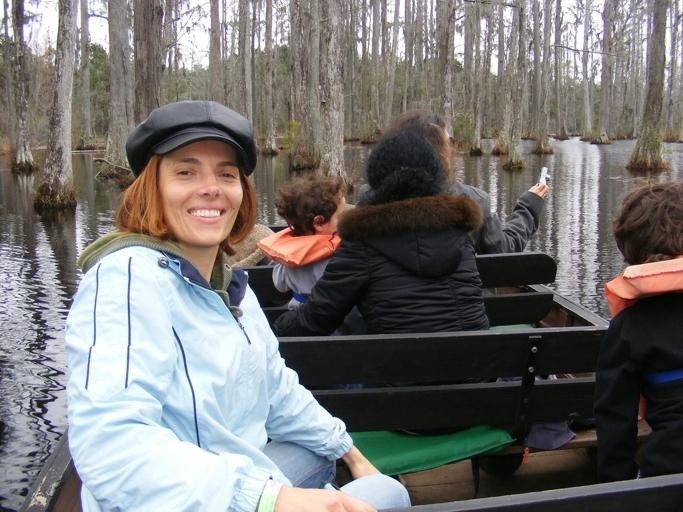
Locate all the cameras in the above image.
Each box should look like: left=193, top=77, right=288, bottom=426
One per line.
left=538, top=166, right=551, bottom=185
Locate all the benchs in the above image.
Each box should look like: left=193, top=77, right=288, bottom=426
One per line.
left=67, top=230, right=683, bottom=511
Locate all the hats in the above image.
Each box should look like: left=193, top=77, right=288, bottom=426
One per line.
left=125, top=99, right=257, bottom=176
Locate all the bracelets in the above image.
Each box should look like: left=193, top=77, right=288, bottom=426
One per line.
left=257, top=479, right=283, bottom=512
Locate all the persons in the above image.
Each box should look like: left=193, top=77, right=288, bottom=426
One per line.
left=64, top=99, right=411, bottom=512
left=593, top=179, right=683, bottom=485
left=256, top=112, right=551, bottom=336
